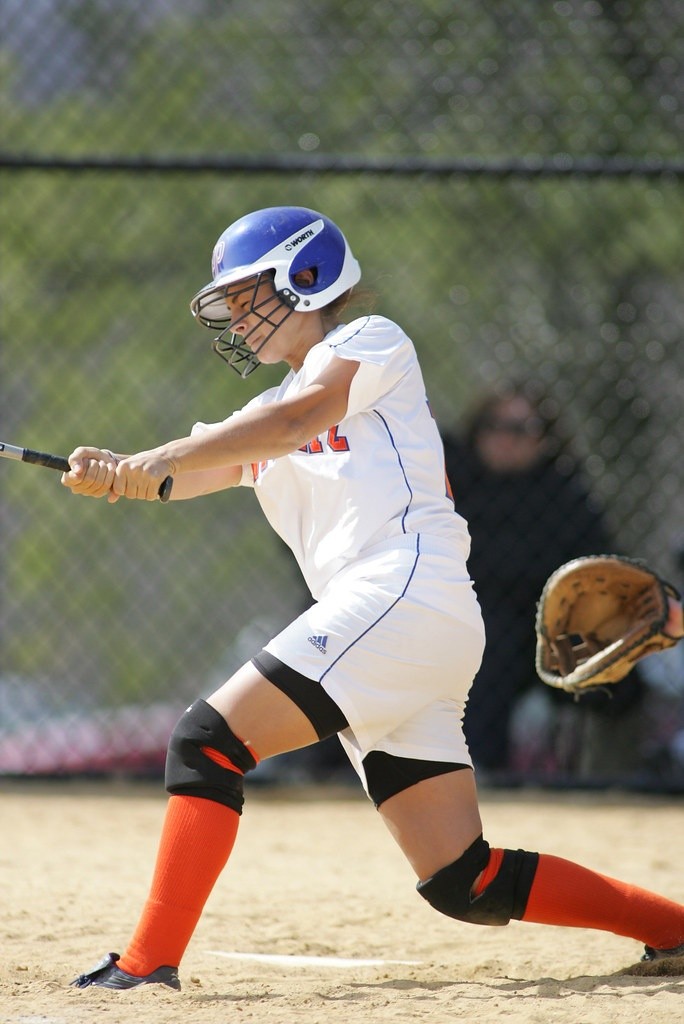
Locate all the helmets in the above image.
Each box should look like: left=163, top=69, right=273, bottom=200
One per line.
left=190, top=206, right=362, bottom=323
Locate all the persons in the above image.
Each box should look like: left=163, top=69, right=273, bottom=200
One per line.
left=425, top=374, right=684, bottom=801
left=60, top=204, right=684, bottom=991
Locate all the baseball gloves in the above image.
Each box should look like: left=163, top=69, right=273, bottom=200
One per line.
left=534, top=554, right=683, bottom=701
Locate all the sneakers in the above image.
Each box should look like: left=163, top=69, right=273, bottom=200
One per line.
left=68, top=952, right=181, bottom=992
left=640, top=942, right=684, bottom=962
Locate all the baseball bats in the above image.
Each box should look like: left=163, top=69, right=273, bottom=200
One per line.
left=1, top=441, right=173, bottom=503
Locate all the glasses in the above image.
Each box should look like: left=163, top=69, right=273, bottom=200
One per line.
left=480, top=416, right=543, bottom=437
